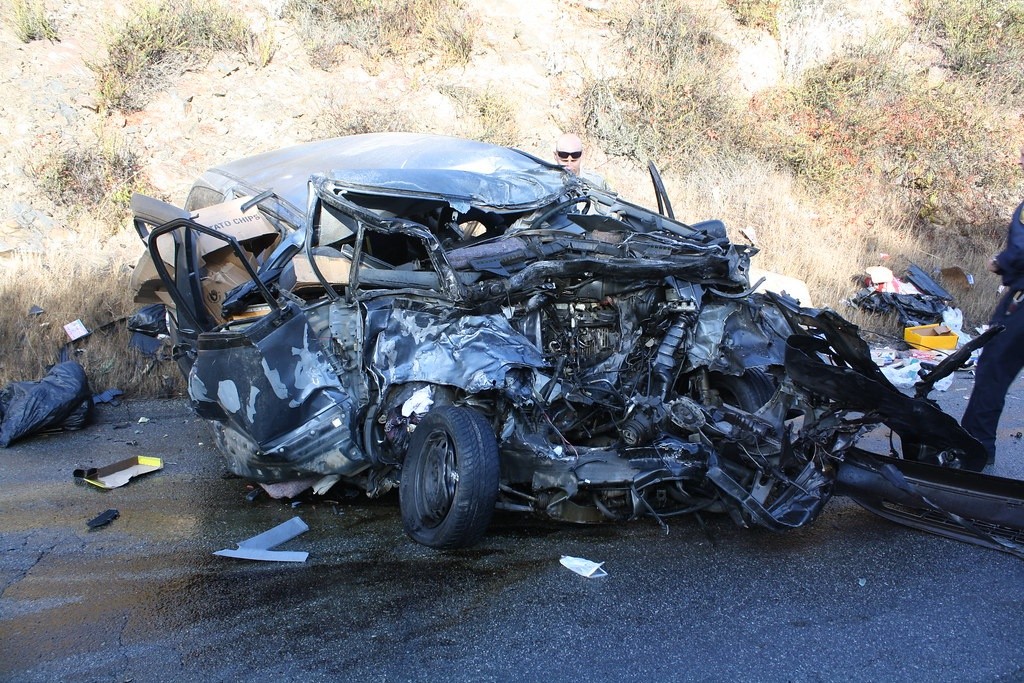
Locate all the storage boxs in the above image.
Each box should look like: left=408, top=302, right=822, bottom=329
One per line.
left=903, top=324, right=959, bottom=350
left=146, top=193, right=280, bottom=273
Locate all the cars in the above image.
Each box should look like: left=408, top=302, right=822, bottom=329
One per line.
left=130, top=132, right=1019, bottom=550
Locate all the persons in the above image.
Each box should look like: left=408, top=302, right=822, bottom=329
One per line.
left=554, top=134, right=622, bottom=216
left=960, top=198, right=1024, bottom=463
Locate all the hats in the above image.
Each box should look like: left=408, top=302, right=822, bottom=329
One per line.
left=556, top=133, right=583, bottom=153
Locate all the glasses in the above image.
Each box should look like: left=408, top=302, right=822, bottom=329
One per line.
left=556, top=152, right=582, bottom=159
